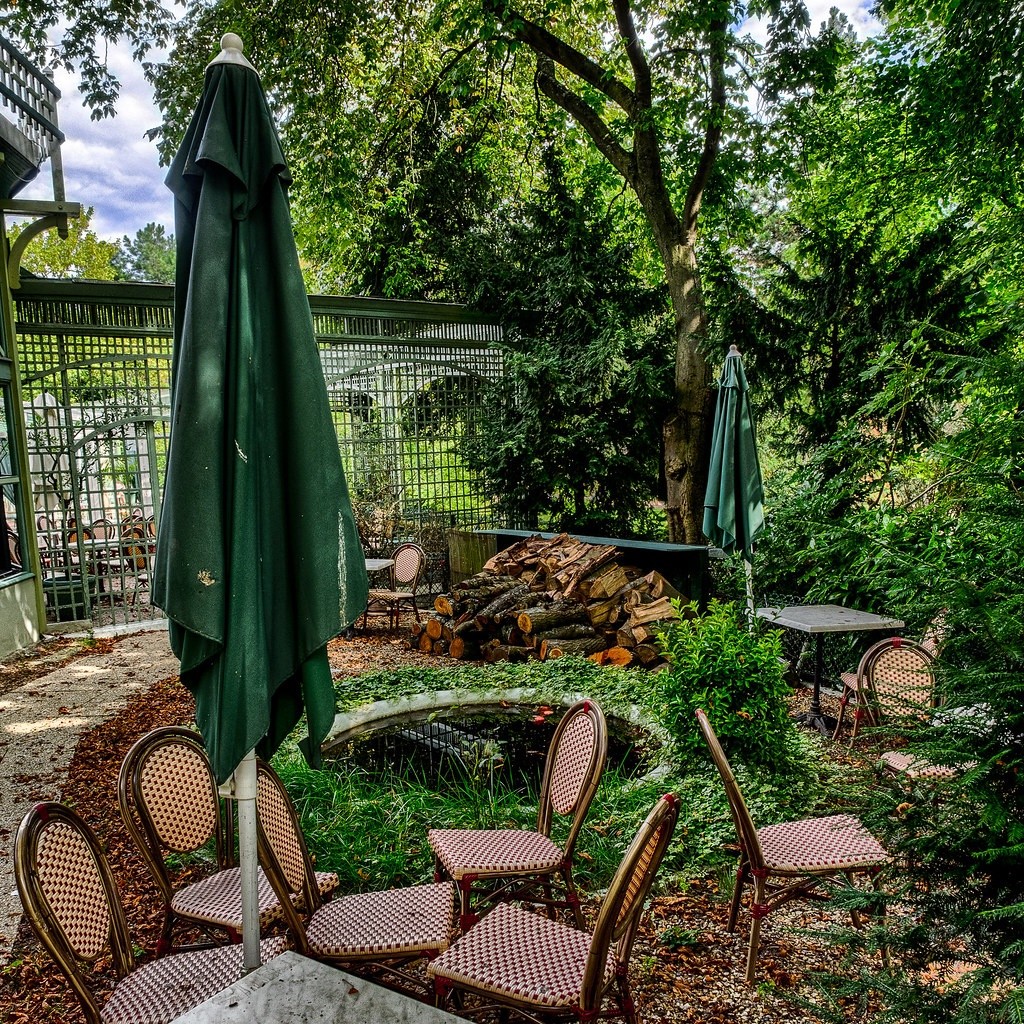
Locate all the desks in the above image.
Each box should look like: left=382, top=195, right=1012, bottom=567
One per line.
left=57, top=537, right=155, bottom=603
left=745, top=604, right=905, bottom=736
left=365, top=558, right=394, bottom=571
left=168, top=950, right=474, bottom=1024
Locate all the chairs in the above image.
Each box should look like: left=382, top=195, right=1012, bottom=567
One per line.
left=255, top=760, right=455, bottom=1007
left=856, top=636, right=979, bottom=871
left=427, top=792, right=682, bottom=1024
left=117, top=726, right=340, bottom=960
left=13, top=801, right=287, bottom=1024
left=694, top=709, right=895, bottom=984
left=428, top=698, right=609, bottom=937
left=364, top=543, right=427, bottom=636
left=832, top=606, right=951, bottom=750
left=7, top=515, right=156, bottom=605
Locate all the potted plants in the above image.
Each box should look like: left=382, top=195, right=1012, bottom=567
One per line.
left=28, top=371, right=156, bottom=620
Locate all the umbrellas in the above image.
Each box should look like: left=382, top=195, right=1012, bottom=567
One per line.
left=149, top=32, right=371, bottom=969
left=702, top=344, right=766, bottom=638
